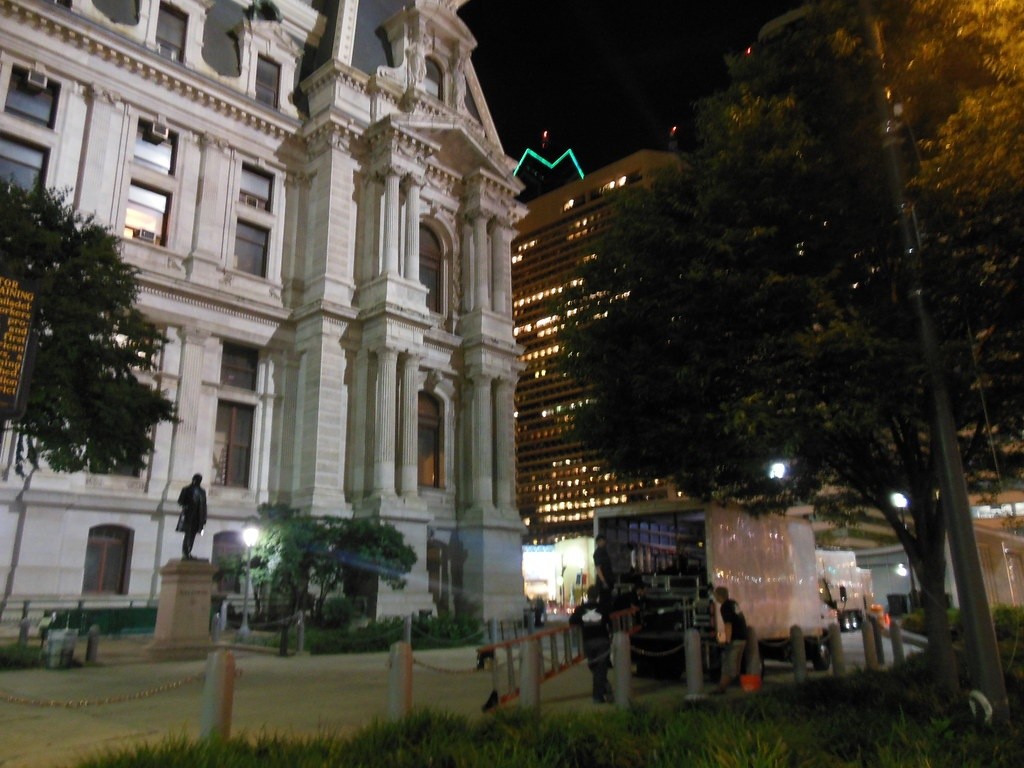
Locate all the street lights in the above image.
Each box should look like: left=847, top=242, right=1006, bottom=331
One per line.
left=236, top=526, right=260, bottom=645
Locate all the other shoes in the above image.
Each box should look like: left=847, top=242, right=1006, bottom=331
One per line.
left=606, top=691, right=614, bottom=704
left=593, top=698, right=606, bottom=704
left=708, top=684, right=724, bottom=694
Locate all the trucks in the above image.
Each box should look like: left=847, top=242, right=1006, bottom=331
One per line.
left=813, top=549, right=875, bottom=634
left=592, top=499, right=847, bottom=676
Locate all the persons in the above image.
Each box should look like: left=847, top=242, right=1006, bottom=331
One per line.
left=589, top=534, right=616, bottom=607
left=533, top=596, right=545, bottom=628
left=569, top=587, right=614, bottom=704
left=693, top=586, right=748, bottom=695
left=177, top=474, right=208, bottom=560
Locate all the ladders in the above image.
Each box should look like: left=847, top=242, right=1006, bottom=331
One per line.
left=474, top=604, right=642, bottom=714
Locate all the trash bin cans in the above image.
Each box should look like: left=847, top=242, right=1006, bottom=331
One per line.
left=886, top=592, right=909, bottom=617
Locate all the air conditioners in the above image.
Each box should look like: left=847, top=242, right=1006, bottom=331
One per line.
left=138, top=228, right=157, bottom=244
left=22, top=69, right=48, bottom=94
left=143, top=121, right=169, bottom=143
left=244, top=195, right=258, bottom=208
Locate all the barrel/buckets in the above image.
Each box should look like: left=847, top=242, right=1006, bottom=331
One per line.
left=740, top=675, right=761, bottom=693
